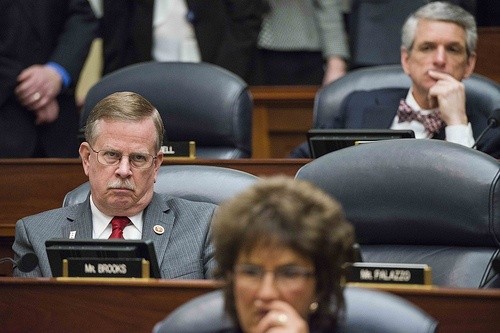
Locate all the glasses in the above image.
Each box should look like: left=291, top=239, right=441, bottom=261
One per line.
left=88, top=144, right=158, bottom=169
left=234, top=258, right=320, bottom=288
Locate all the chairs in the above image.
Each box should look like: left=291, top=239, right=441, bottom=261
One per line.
left=313, top=65, right=500, bottom=129
left=63, top=165, right=265, bottom=206
left=294, top=139, right=500, bottom=289
left=81, top=61, right=252, bottom=159
left=153, top=288, right=439, bottom=333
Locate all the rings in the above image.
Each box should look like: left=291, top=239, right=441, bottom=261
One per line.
left=32, top=92, right=41, bottom=102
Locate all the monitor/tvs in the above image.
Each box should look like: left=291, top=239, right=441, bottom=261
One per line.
left=305, top=128, right=415, bottom=160
left=45, top=238, right=162, bottom=280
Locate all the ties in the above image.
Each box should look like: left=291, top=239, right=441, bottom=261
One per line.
left=107, top=216, right=131, bottom=240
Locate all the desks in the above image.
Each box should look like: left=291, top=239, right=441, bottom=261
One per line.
left=0, top=85, right=500, bottom=333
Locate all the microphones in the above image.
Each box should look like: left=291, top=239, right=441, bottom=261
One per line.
left=0, top=253, right=39, bottom=273
left=470, top=108, right=500, bottom=148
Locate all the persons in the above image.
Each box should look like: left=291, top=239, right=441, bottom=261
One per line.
left=256, top=0, right=350, bottom=87
left=12, top=91, right=224, bottom=282
left=100, top=0, right=271, bottom=82
left=191, top=172, right=359, bottom=333
left=0, top=0, right=96, bottom=158
left=286, top=1, right=500, bottom=162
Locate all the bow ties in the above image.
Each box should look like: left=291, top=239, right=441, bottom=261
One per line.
left=396, top=98, right=446, bottom=134
